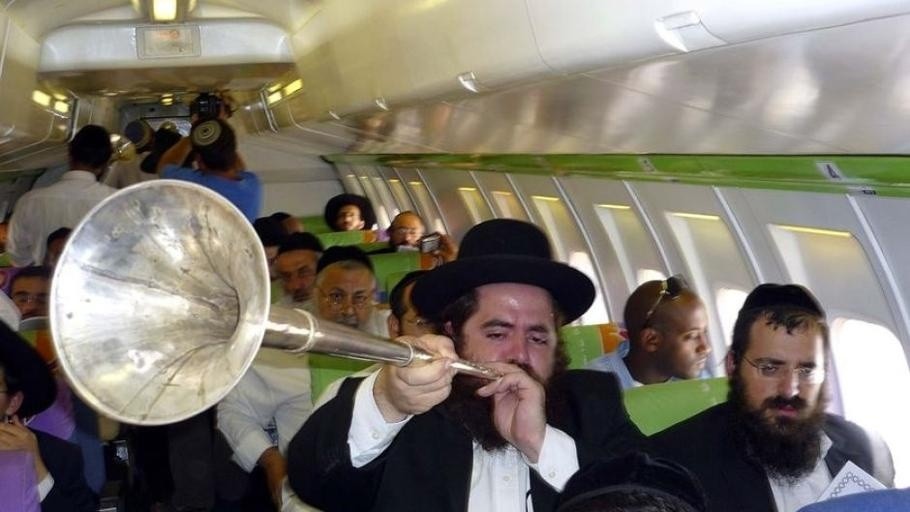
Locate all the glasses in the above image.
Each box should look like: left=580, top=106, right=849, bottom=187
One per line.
left=740, top=354, right=826, bottom=384
left=642, top=273, right=689, bottom=325
left=316, top=284, right=378, bottom=312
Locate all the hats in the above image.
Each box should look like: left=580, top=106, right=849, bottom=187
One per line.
left=324, top=193, right=377, bottom=231
left=316, top=245, right=374, bottom=273
left=0, top=318, right=57, bottom=419
left=408, top=218, right=596, bottom=329
left=278, top=232, right=323, bottom=254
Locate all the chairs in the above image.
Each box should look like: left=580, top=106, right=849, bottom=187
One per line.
left=266, top=215, right=736, bottom=441
left=16, top=325, right=55, bottom=361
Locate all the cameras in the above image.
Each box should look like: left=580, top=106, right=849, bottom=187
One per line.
left=198, top=92, right=218, bottom=118
left=420, top=233, right=440, bottom=254
